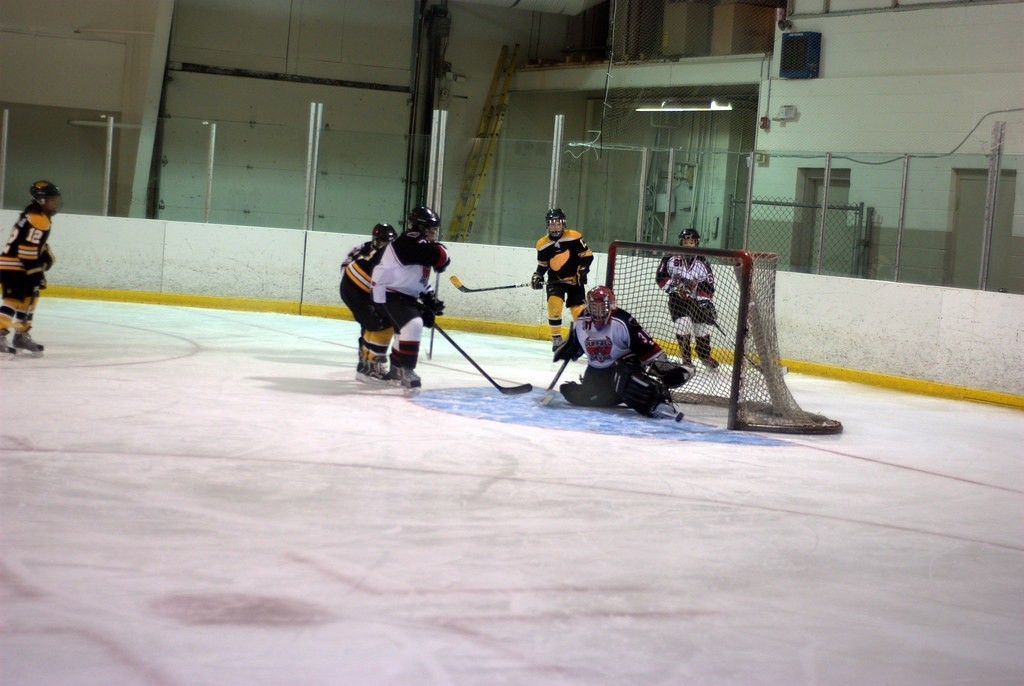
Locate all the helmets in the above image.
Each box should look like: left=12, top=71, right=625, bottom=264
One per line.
left=586, top=285, right=616, bottom=329
left=30, top=181, right=61, bottom=216
left=545, top=208, right=567, bottom=236
left=409, top=207, right=441, bottom=242
left=372, top=223, right=397, bottom=248
left=679, top=229, right=699, bottom=246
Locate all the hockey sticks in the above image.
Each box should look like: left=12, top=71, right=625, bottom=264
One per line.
left=433, top=323, right=533, bottom=396
left=449, top=275, right=573, bottom=293
left=1, top=262, right=47, bottom=328
left=689, top=296, right=788, bottom=380
left=539, top=359, right=571, bottom=406
left=424, top=271, right=440, bottom=360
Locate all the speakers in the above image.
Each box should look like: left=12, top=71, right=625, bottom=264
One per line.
left=779, top=31, right=821, bottom=78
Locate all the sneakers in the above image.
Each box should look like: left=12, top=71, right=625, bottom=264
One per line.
left=703, top=357, right=719, bottom=371
left=553, top=335, right=562, bottom=352
left=390, top=364, right=402, bottom=386
left=400, top=367, right=422, bottom=396
left=12, top=326, right=43, bottom=357
left=0, top=329, right=16, bottom=360
left=356, top=351, right=390, bottom=385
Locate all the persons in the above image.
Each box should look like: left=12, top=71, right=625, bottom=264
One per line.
left=370, top=207, right=451, bottom=388
left=553, top=287, right=695, bottom=422
left=532, top=207, right=595, bottom=351
left=340, top=223, right=445, bottom=384
left=656, top=228, right=718, bottom=372
left=0, top=180, right=62, bottom=357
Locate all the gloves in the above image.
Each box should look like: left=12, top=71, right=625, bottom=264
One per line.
left=41, top=250, right=54, bottom=270
left=576, top=266, right=589, bottom=286
left=417, top=303, right=435, bottom=328
left=670, top=278, right=693, bottom=299
left=419, top=288, right=445, bottom=316
left=531, top=272, right=544, bottom=289
left=699, top=301, right=717, bottom=323
left=29, top=270, right=47, bottom=291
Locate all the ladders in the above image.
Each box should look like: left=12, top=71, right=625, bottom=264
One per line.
left=445, top=41, right=520, bottom=243
